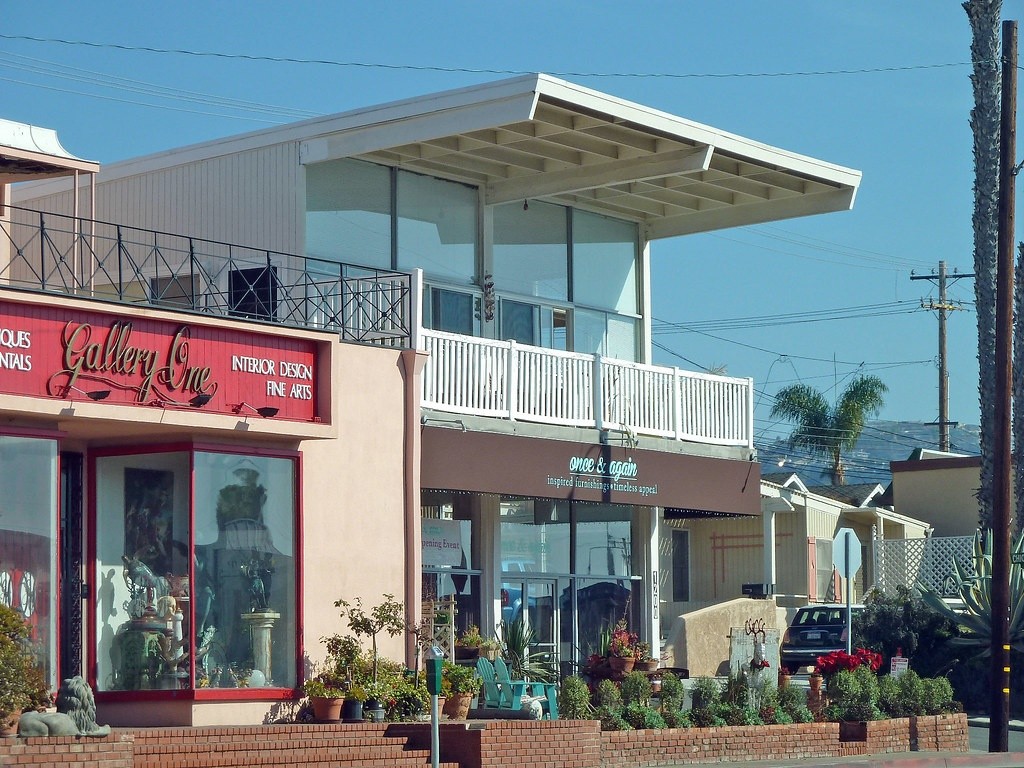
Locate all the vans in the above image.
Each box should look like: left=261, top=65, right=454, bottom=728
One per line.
left=463, top=559, right=571, bottom=643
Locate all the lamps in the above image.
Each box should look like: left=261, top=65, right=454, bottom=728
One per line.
left=751, top=452, right=792, bottom=467
left=156, top=395, right=212, bottom=408
left=422, top=415, right=467, bottom=434
left=601, top=435, right=639, bottom=446
left=234, top=402, right=279, bottom=417
left=61, top=385, right=111, bottom=401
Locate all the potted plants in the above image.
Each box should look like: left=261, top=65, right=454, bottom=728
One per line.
left=608, top=592, right=635, bottom=676
left=0, top=603, right=53, bottom=738
left=442, top=658, right=483, bottom=721
left=341, top=688, right=370, bottom=720
left=651, top=674, right=661, bottom=692
left=364, top=680, right=395, bottom=710
left=635, top=643, right=659, bottom=671
left=299, top=679, right=346, bottom=719
left=419, top=671, right=453, bottom=721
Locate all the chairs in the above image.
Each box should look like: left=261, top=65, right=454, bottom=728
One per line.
left=476, top=657, right=547, bottom=721
left=817, top=614, right=829, bottom=624
left=492, top=656, right=558, bottom=720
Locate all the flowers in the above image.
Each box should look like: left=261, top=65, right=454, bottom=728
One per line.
left=778, top=667, right=790, bottom=675
left=811, top=666, right=823, bottom=678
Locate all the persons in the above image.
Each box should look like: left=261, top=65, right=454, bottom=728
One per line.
left=247, top=546, right=267, bottom=609
left=157, top=596, right=177, bottom=620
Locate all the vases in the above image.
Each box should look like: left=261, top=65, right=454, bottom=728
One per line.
left=370, top=709, right=386, bottom=722
left=778, top=675, right=790, bottom=688
left=809, top=677, right=823, bottom=691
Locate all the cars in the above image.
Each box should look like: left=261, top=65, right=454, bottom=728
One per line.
left=779, top=604, right=866, bottom=674
